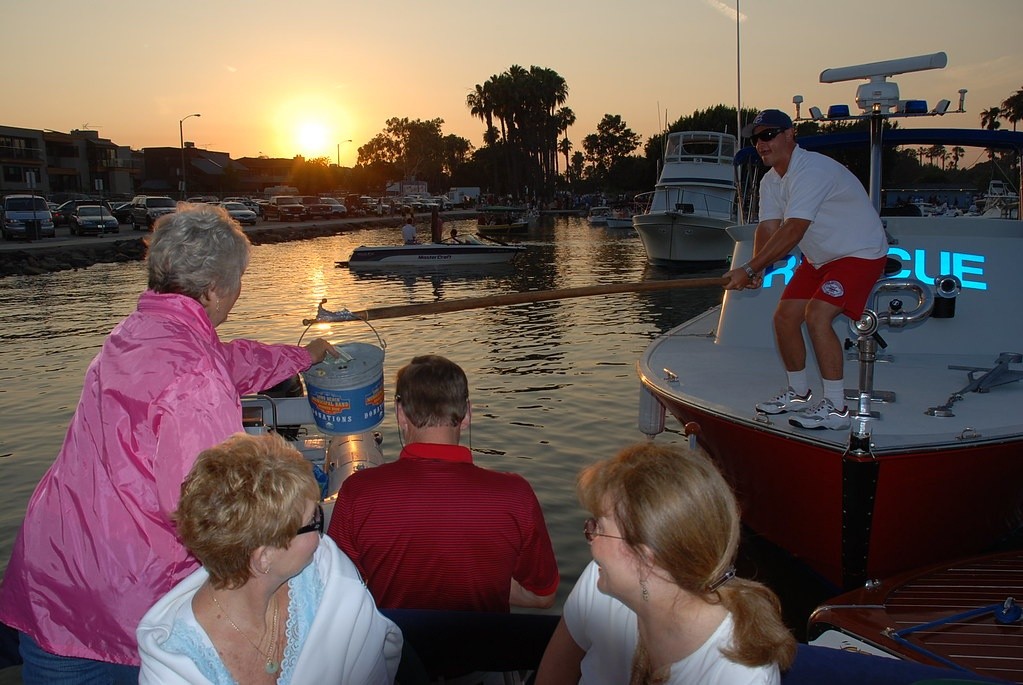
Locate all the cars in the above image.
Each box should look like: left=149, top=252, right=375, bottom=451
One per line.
left=217, top=202, right=257, bottom=226
left=185, top=195, right=265, bottom=217
left=47, top=201, right=60, bottom=211
left=109, top=200, right=132, bottom=224
left=68, top=205, right=119, bottom=236
left=297, top=193, right=454, bottom=220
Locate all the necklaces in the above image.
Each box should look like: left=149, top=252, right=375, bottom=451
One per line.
left=210, top=582, right=279, bottom=674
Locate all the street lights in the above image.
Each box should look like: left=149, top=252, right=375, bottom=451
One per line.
left=180, top=112, right=201, bottom=200
left=337, top=139, right=352, bottom=166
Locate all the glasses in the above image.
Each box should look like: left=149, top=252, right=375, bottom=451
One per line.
left=582, top=519, right=630, bottom=545
left=749, top=126, right=787, bottom=147
left=292, top=504, right=325, bottom=538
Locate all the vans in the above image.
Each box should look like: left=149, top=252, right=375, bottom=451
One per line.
left=0, top=194, right=56, bottom=241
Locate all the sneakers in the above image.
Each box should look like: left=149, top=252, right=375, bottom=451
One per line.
left=787, top=399, right=851, bottom=429
left=754, top=385, right=812, bottom=415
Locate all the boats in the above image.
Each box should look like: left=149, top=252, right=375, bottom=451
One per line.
left=606, top=210, right=635, bottom=228
left=586, top=206, right=612, bottom=224
left=347, top=231, right=528, bottom=268
left=633, top=100, right=747, bottom=272
left=910, top=178, right=1019, bottom=220
left=475, top=205, right=530, bottom=237
left=636, top=0, right=1023, bottom=592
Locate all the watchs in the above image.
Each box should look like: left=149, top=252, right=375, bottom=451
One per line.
left=743, top=264, right=756, bottom=280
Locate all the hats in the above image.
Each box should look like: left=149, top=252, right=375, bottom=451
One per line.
left=741, top=110, right=793, bottom=138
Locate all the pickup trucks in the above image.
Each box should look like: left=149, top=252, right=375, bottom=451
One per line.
left=258, top=195, right=306, bottom=223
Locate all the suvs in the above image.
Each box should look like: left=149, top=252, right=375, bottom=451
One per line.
left=51, top=199, right=112, bottom=228
left=130, top=194, right=177, bottom=232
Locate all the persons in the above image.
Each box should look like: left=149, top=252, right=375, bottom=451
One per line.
left=537, top=440, right=796, bottom=685
left=896, top=195, right=923, bottom=207
left=574, top=195, right=579, bottom=209
left=390, top=200, right=396, bottom=218
left=723, top=109, right=889, bottom=431
left=402, top=218, right=417, bottom=245
left=928, top=194, right=960, bottom=206
left=585, top=196, right=589, bottom=206
left=136, top=432, right=404, bottom=685
left=0, top=201, right=339, bottom=685
left=451, top=229, right=463, bottom=244
left=377, top=198, right=382, bottom=218
left=328, top=355, right=561, bottom=685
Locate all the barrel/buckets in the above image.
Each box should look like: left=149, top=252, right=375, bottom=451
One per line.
left=296, top=311, right=386, bottom=437
